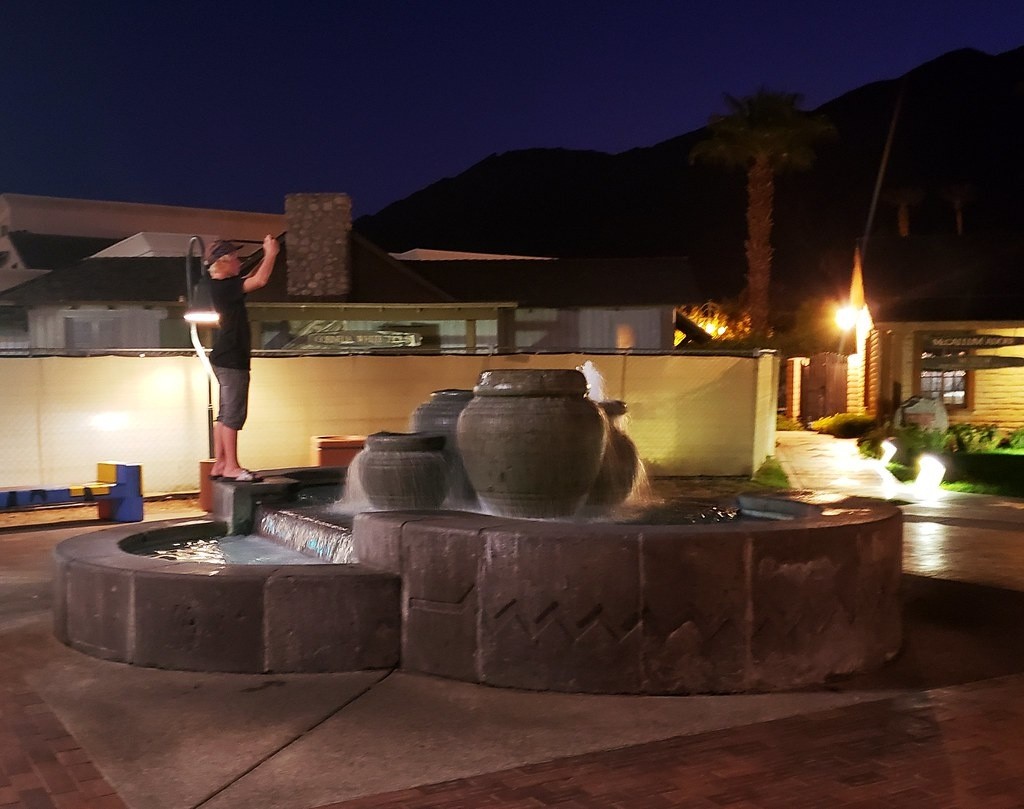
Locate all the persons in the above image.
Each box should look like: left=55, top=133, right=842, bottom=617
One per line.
left=205, top=234, right=280, bottom=483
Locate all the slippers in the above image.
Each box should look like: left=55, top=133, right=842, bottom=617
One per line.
left=218, top=469, right=266, bottom=483
left=208, top=474, right=223, bottom=479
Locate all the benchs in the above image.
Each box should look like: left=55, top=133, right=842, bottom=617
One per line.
left=0, top=462, right=143, bottom=522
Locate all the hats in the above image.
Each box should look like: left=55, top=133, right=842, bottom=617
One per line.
left=203, top=239, right=245, bottom=266
